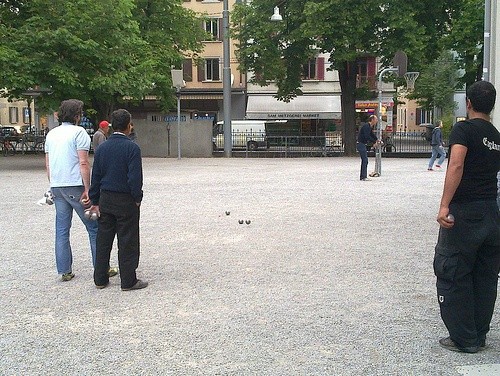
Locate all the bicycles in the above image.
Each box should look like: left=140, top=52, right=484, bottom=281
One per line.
left=383, top=137, right=397, bottom=153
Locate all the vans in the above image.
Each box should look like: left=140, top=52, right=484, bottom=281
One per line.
left=212, top=120, right=268, bottom=151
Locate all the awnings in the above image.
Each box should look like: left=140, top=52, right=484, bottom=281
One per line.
left=245, top=94, right=341, bottom=120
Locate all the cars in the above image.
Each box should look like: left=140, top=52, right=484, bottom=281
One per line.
left=0, top=125, right=21, bottom=143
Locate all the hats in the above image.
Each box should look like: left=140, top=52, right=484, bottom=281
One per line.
left=99, top=120, right=109, bottom=128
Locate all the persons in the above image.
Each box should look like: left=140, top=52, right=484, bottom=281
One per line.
left=433, top=81, right=500, bottom=353
left=357, top=115, right=383, bottom=181
left=44, top=98, right=119, bottom=282
left=127, top=123, right=137, bottom=142
left=84, top=109, right=148, bottom=291
left=428, top=121, right=446, bottom=171
left=93, top=121, right=111, bottom=154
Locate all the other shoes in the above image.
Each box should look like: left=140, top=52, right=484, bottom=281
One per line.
left=428, top=169, right=433, bottom=170
left=109, top=267, right=119, bottom=276
left=96, top=280, right=109, bottom=288
left=122, top=279, right=149, bottom=290
left=439, top=336, right=486, bottom=353
left=362, top=178, right=372, bottom=181
left=436, top=165, right=441, bottom=168
left=62, top=272, right=75, bottom=281
